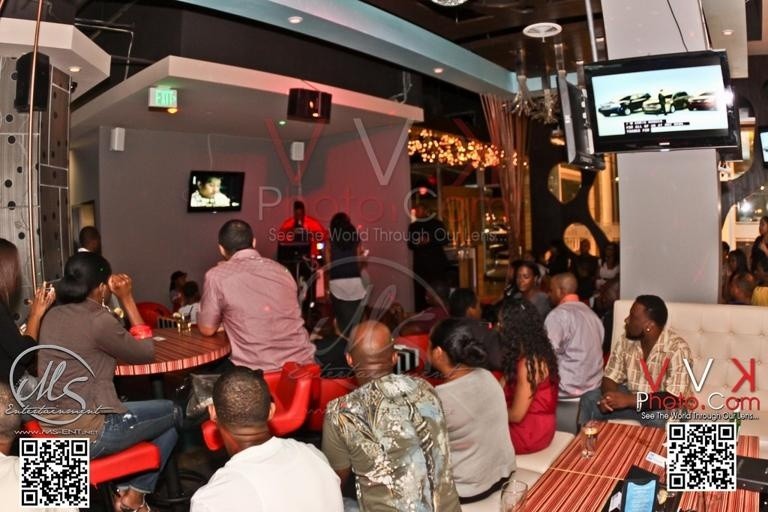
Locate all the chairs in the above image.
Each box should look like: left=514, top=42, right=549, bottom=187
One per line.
left=390, top=337, right=431, bottom=371
left=121, top=301, right=172, bottom=330
left=307, top=376, right=357, bottom=433
left=21, top=418, right=161, bottom=512
left=200, top=361, right=311, bottom=451
left=262, top=364, right=320, bottom=398
left=750, top=286, right=768, bottom=308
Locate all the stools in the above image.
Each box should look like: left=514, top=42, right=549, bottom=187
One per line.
left=510, top=432, right=577, bottom=473
left=458, top=468, right=540, bottom=512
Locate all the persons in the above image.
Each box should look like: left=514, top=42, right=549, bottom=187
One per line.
left=751, top=217, right=768, bottom=276
left=169, top=271, right=189, bottom=326
left=274, top=201, right=329, bottom=323
left=0, top=237, right=56, bottom=457
left=590, top=242, right=619, bottom=352
left=750, top=254, right=768, bottom=307
left=408, top=204, right=462, bottom=314
left=77, top=227, right=102, bottom=253
left=722, top=241, right=733, bottom=304
left=727, top=248, right=758, bottom=305
left=542, top=272, right=606, bottom=403
left=449, top=289, right=505, bottom=372
left=576, top=294, right=694, bottom=434
left=425, top=319, right=516, bottom=505
left=175, top=220, right=316, bottom=445
left=492, top=299, right=559, bottom=454
left=321, top=320, right=463, bottom=511
left=569, top=239, right=599, bottom=304
left=180, top=282, right=200, bottom=326
left=38, top=252, right=183, bottom=512
left=189, top=366, right=347, bottom=512
left=191, top=174, right=241, bottom=207
left=505, top=261, right=555, bottom=322
left=325, top=212, right=372, bottom=369
left=1, top=382, right=79, bottom=512
left=525, top=249, right=549, bottom=287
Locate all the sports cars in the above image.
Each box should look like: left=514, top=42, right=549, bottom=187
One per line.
left=598, top=92, right=651, bottom=117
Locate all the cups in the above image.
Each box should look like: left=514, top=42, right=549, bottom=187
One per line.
left=499, top=479, right=528, bottom=512
left=579, top=419, right=601, bottom=458
left=175, top=313, right=193, bottom=335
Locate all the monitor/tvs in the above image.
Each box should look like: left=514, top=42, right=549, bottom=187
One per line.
left=578, top=50, right=738, bottom=154
left=186, top=169, right=246, bottom=213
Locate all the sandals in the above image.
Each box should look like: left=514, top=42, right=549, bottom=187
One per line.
left=120, top=495, right=152, bottom=512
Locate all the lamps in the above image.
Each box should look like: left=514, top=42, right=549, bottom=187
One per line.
left=523, top=5, right=562, bottom=39
left=547, top=125, right=567, bottom=148
left=408, top=129, right=529, bottom=171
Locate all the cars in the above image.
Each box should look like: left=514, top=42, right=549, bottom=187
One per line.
left=642, top=91, right=689, bottom=113
left=687, top=93, right=718, bottom=110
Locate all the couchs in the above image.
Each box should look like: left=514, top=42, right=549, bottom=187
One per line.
left=611, top=297, right=768, bottom=461
